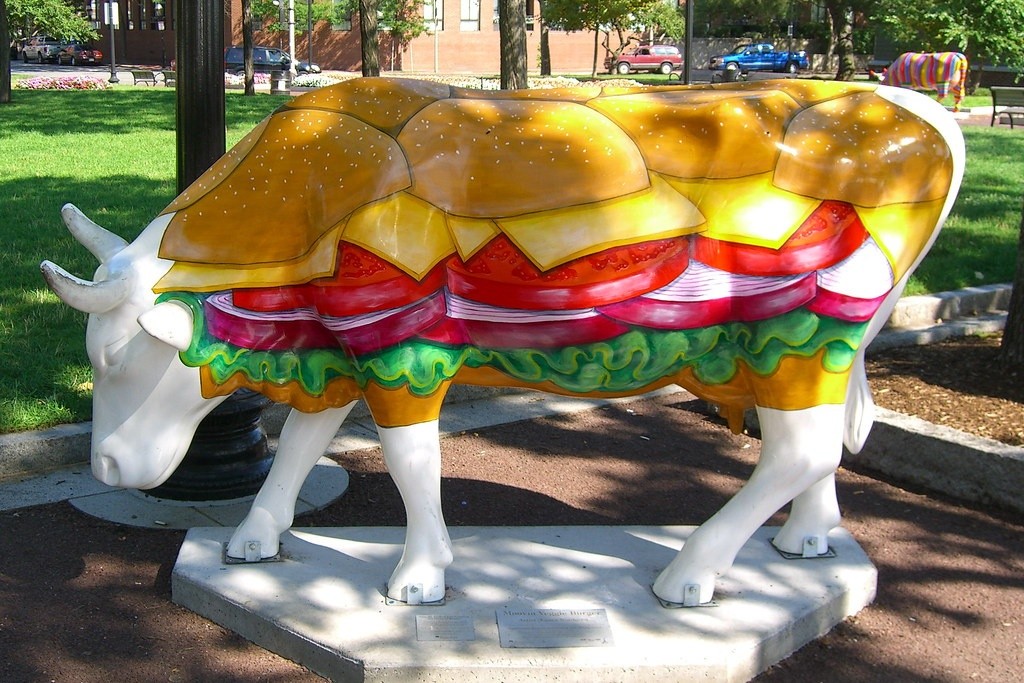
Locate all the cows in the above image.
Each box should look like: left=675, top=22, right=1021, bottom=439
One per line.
left=39, top=73, right=967, bottom=611
left=870, top=51, right=969, bottom=113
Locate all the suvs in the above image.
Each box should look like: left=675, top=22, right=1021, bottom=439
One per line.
left=224, top=44, right=322, bottom=76
left=604, top=44, right=684, bottom=75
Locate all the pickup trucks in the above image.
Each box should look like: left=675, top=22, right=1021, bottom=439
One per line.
left=21, top=34, right=69, bottom=65
left=708, top=42, right=812, bottom=77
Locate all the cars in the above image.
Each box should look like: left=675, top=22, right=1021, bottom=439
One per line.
left=56, top=44, right=103, bottom=67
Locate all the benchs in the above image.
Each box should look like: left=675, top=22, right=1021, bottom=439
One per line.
left=130, top=70, right=160, bottom=86
left=737, top=71, right=798, bottom=81
left=712, top=70, right=741, bottom=83
left=162, top=71, right=176, bottom=87
left=990, top=86, right=1024, bottom=129
left=668, top=70, right=723, bottom=85
